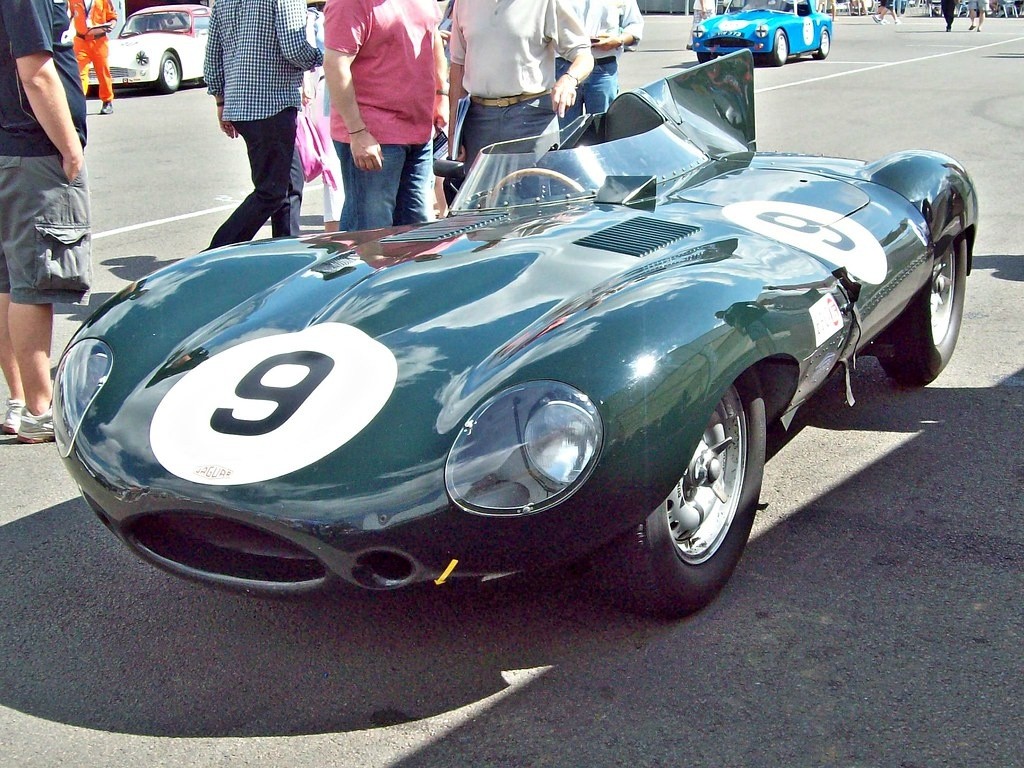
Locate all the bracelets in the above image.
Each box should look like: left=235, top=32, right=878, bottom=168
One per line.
left=348, top=126, right=367, bottom=134
left=563, top=72, right=579, bottom=89
left=216, top=102, right=225, bottom=106
left=616, top=36, right=624, bottom=51
left=436, top=89, right=449, bottom=96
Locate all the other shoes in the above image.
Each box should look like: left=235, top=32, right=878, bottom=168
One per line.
left=686, top=44, right=695, bottom=49
left=968, top=25, right=976, bottom=30
left=977, top=27, right=981, bottom=32
left=872, top=16, right=881, bottom=24
left=946, top=27, right=951, bottom=33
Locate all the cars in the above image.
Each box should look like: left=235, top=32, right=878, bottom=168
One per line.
left=51, top=51, right=975, bottom=622
left=86, top=4, right=213, bottom=95
left=687, top=0, right=833, bottom=68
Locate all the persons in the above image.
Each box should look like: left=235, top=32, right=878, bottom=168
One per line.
left=687, top=0, right=716, bottom=50
left=0, top=0, right=92, bottom=444
left=198, top=0, right=644, bottom=252
left=817, top=0, right=1024, bottom=32
left=68, top=0, right=119, bottom=114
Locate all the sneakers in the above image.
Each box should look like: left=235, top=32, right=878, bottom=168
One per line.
left=17, top=406, right=57, bottom=444
left=101, top=101, right=113, bottom=114
left=2, top=397, right=28, bottom=435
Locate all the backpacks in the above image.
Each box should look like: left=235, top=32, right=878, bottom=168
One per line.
left=296, top=105, right=338, bottom=192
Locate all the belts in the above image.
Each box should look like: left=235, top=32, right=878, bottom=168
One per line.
left=76, top=31, right=106, bottom=39
left=594, top=56, right=616, bottom=66
left=471, top=89, right=552, bottom=108
